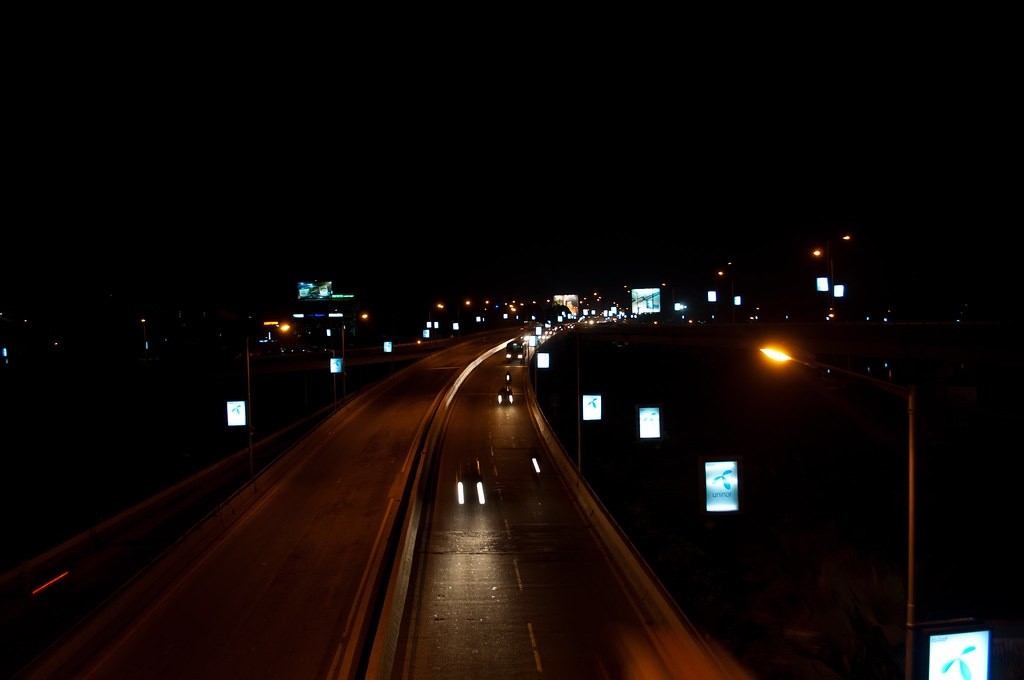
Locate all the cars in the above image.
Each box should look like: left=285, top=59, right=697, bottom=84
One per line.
left=497, top=388, right=514, bottom=406
left=453, top=456, right=489, bottom=508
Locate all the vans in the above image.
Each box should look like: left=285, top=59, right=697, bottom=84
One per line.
left=506, top=341, right=523, bottom=360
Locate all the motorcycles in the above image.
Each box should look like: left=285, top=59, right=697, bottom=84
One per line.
left=505, top=374, right=512, bottom=384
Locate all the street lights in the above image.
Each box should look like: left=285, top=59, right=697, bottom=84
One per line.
left=760, top=342, right=917, bottom=680
left=812, top=236, right=851, bottom=320
left=429, top=304, right=444, bottom=350
left=246, top=324, right=290, bottom=493
left=457, top=301, right=470, bottom=334
left=662, top=283, right=675, bottom=315
left=718, top=262, right=736, bottom=325
left=342, top=313, right=368, bottom=396
